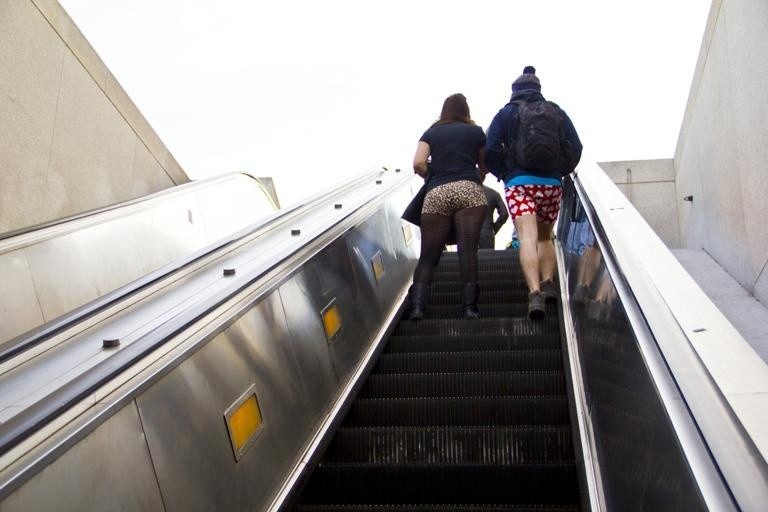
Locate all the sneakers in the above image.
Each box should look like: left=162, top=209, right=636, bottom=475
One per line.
left=540, top=278, right=557, bottom=298
left=527, top=290, right=546, bottom=318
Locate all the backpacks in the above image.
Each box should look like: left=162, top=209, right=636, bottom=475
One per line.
left=507, top=101, right=562, bottom=172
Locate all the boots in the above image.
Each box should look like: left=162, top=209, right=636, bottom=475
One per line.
left=463, top=281, right=481, bottom=320
left=408, top=283, right=429, bottom=319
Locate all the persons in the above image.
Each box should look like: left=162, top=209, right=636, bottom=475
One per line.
left=478, top=173, right=508, bottom=251
left=484, top=67, right=583, bottom=320
left=408, top=94, right=487, bottom=319
left=567, top=218, right=618, bottom=327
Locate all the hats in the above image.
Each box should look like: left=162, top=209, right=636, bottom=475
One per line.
left=511, top=66, right=541, bottom=91
